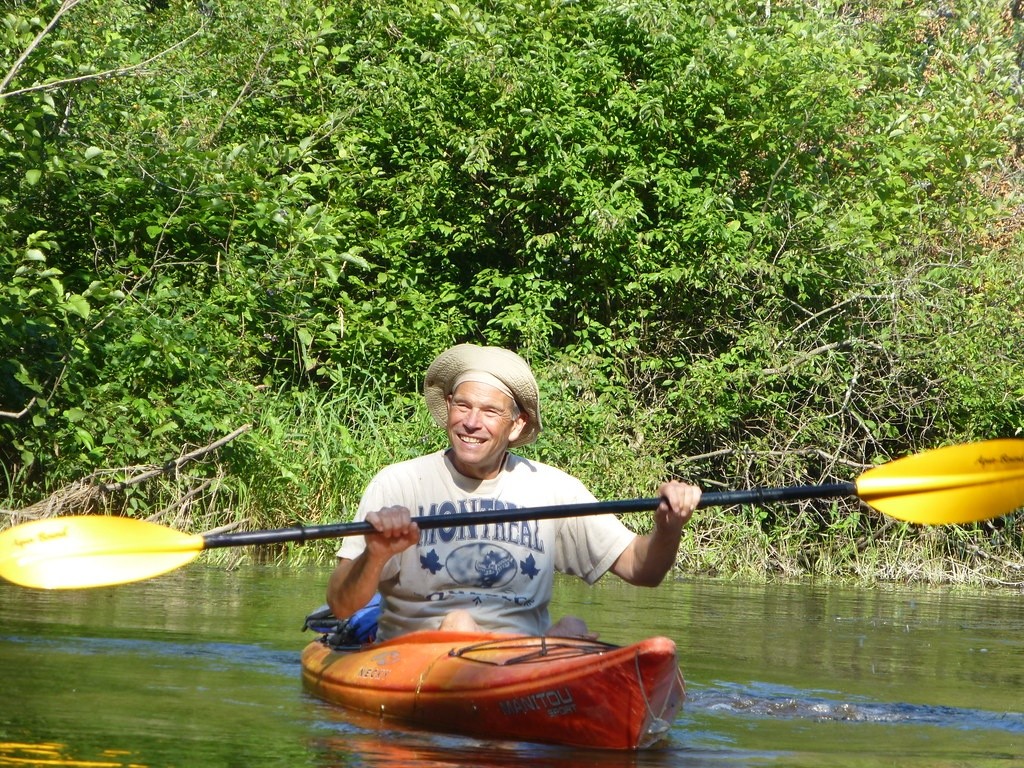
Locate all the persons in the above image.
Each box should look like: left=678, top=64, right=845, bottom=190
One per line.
left=326, top=344, right=702, bottom=649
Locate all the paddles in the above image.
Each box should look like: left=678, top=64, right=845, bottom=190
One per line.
left=0, top=436, right=1023, bottom=591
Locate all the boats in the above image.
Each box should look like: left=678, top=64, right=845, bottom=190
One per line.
left=302, top=626, right=687, bottom=751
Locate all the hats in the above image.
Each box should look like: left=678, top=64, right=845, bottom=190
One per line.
left=424, top=344, right=544, bottom=448
